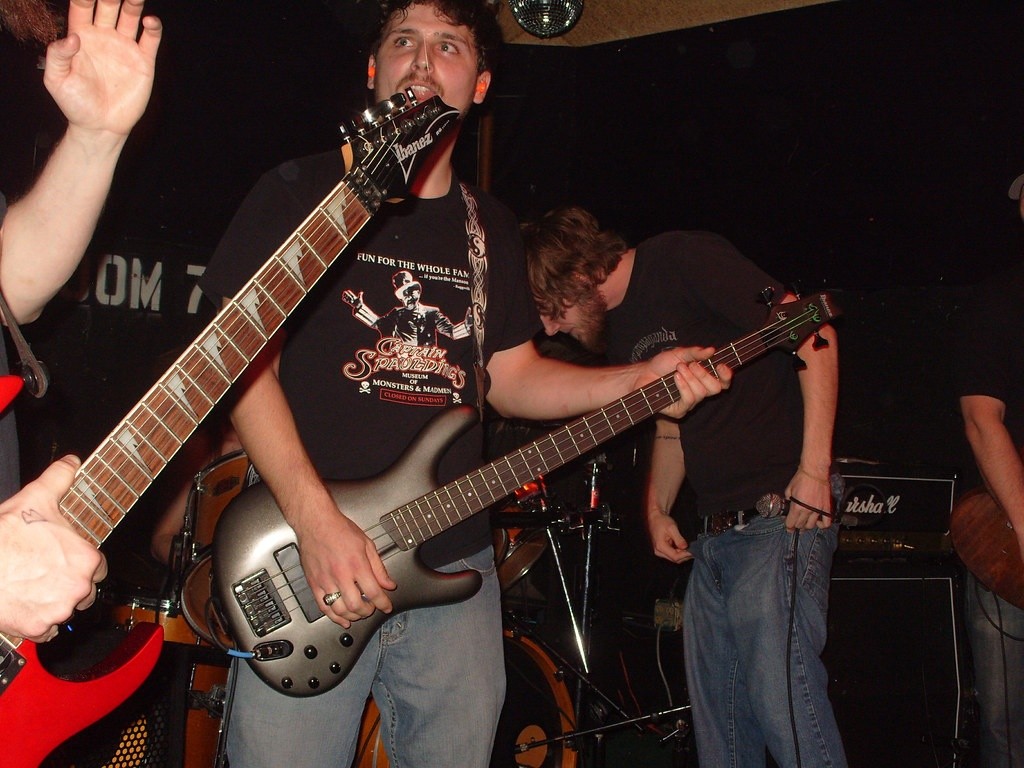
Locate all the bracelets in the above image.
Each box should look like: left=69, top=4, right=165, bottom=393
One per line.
left=790, top=496, right=833, bottom=519
left=798, top=465, right=831, bottom=488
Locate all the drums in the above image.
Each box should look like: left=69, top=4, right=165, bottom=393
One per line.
left=66, top=587, right=233, bottom=768
left=494, top=498, right=549, bottom=593
left=357, top=626, right=578, bottom=768
left=178, top=447, right=252, bottom=652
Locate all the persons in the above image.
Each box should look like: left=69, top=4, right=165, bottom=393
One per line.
left=520, top=205, right=850, bottom=768
left=949, top=171, right=1024, bottom=768
left=197, top=0, right=732, bottom=768
left=0, top=0, right=162, bottom=643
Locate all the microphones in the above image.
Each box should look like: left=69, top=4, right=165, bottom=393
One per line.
left=754, top=491, right=857, bottom=529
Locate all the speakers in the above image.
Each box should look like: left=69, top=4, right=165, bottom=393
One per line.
left=2, top=237, right=235, bottom=768
left=818, top=557, right=979, bottom=768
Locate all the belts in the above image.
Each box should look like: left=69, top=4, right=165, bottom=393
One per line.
left=699, top=508, right=759, bottom=537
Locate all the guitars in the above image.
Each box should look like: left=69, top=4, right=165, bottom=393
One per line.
left=210, top=273, right=858, bottom=699
left=948, top=447, right=1023, bottom=612
left=0, top=86, right=465, bottom=768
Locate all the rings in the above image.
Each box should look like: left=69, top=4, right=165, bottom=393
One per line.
left=323, top=590, right=341, bottom=606
